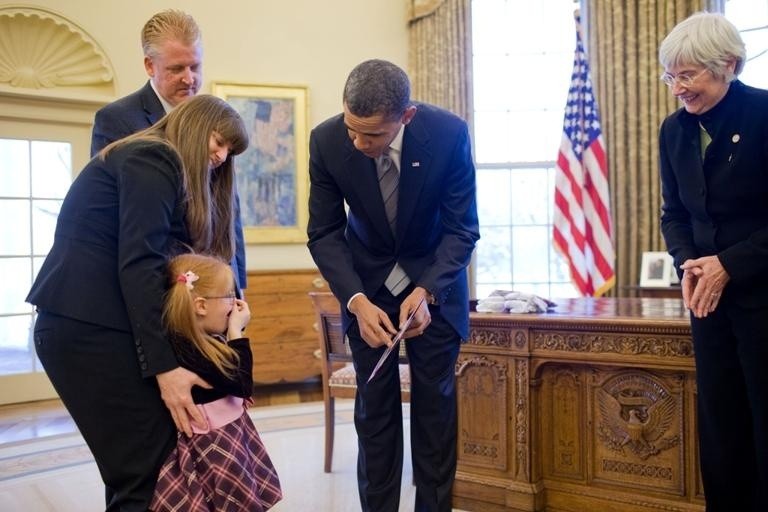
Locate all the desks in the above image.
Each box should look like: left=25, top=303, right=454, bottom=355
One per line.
left=452, top=296, right=708, bottom=512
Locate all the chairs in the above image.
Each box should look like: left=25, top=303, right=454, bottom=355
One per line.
left=308, top=291, right=411, bottom=473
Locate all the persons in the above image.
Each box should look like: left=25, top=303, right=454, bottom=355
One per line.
left=653, top=11, right=768, bottom=512
left=89, top=9, right=247, bottom=299
left=24, top=93, right=249, bottom=512
left=305, top=59, right=481, bottom=512
left=148, top=254, right=283, bottom=512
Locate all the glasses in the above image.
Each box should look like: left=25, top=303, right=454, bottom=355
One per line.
left=200, top=290, right=240, bottom=301
left=658, top=62, right=718, bottom=87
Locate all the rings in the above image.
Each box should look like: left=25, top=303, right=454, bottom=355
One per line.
left=710, top=292, right=718, bottom=297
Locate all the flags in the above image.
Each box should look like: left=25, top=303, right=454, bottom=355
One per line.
left=550, top=6, right=617, bottom=298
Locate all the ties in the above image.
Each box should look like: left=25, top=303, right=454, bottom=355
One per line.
left=376, top=152, right=404, bottom=247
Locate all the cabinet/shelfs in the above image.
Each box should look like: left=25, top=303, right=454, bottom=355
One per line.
left=242, top=269, right=331, bottom=386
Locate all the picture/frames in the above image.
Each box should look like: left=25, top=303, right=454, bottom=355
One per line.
left=640, top=251, right=670, bottom=287
left=212, top=81, right=310, bottom=246
